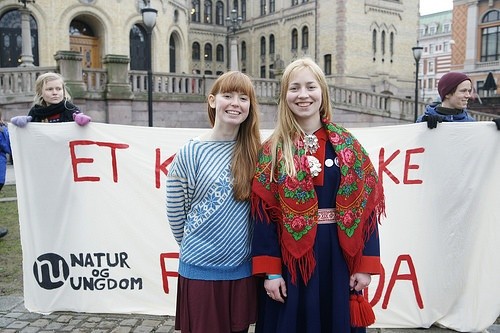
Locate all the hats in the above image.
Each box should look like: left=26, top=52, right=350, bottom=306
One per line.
left=438, top=72, right=471, bottom=102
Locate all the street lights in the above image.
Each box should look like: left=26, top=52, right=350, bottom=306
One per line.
left=412, top=40, right=423, bottom=124
left=224, top=8, right=243, bottom=74
left=140, top=0, right=158, bottom=128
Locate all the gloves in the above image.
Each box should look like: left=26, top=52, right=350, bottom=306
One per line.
left=72, top=113, right=92, bottom=126
left=420, top=112, right=443, bottom=128
left=11, top=114, right=33, bottom=127
left=491, top=117, right=500, bottom=129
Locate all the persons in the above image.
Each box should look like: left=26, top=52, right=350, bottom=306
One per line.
left=164, top=69, right=265, bottom=333
left=415, top=70, right=500, bottom=131
left=249, top=57, right=385, bottom=332
left=9, top=71, right=94, bottom=127
left=0, top=111, right=16, bottom=236
